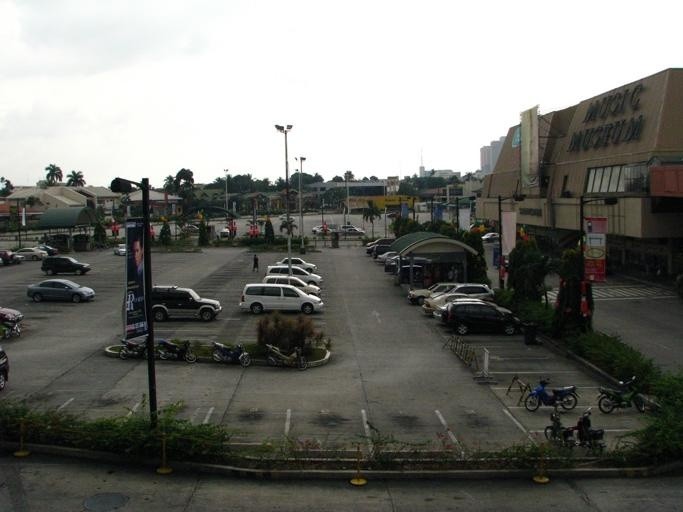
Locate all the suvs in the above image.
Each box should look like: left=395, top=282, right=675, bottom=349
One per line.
left=445, top=300, right=521, bottom=336
left=429, top=283, right=495, bottom=304
left=0, top=347, right=10, bottom=391
left=149, top=284, right=221, bottom=323
left=39, top=256, right=91, bottom=276
left=406, top=283, right=456, bottom=306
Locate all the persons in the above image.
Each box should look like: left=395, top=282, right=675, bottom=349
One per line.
left=127, top=239, right=144, bottom=286
left=252, top=254, right=259, bottom=273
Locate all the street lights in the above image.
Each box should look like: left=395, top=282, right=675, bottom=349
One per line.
left=274, top=123, right=293, bottom=276
left=397, top=196, right=410, bottom=219
left=430, top=197, right=446, bottom=225
left=579, top=194, right=618, bottom=283
left=497, top=193, right=526, bottom=290
left=110, top=175, right=157, bottom=431
left=222, top=169, right=228, bottom=218
left=455, top=195, right=475, bottom=232
left=299, top=157, right=306, bottom=238
left=16, top=197, right=25, bottom=248
left=294, top=169, right=300, bottom=237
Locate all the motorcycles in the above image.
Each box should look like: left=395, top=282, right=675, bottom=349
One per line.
left=157, top=338, right=197, bottom=364
left=212, top=338, right=251, bottom=367
left=577, top=407, right=606, bottom=456
left=265, top=342, right=308, bottom=370
left=3, top=314, right=21, bottom=339
left=595, top=375, right=646, bottom=414
left=544, top=403, right=576, bottom=449
left=524, top=376, right=580, bottom=411
left=117, top=334, right=148, bottom=361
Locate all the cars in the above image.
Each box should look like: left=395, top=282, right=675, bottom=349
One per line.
left=421, top=292, right=469, bottom=314
left=431, top=298, right=512, bottom=322
left=481, top=231, right=498, bottom=243
left=387, top=211, right=397, bottom=218
left=274, top=258, right=317, bottom=275
left=365, top=235, right=431, bottom=278
left=26, top=278, right=96, bottom=304
left=112, top=242, right=126, bottom=257
left=0, top=306, right=22, bottom=326
left=182, top=225, right=200, bottom=234
left=311, top=223, right=365, bottom=237
left=219, top=227, right=229, bottom=237
left=0, top=244, right=58, bottom=265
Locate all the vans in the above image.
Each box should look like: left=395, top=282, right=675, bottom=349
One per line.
left=264, top=265, right=322, bottom=286
left=262, top=275, right=322, bottom=297
left=238, top=282, right=323, bottom=315
